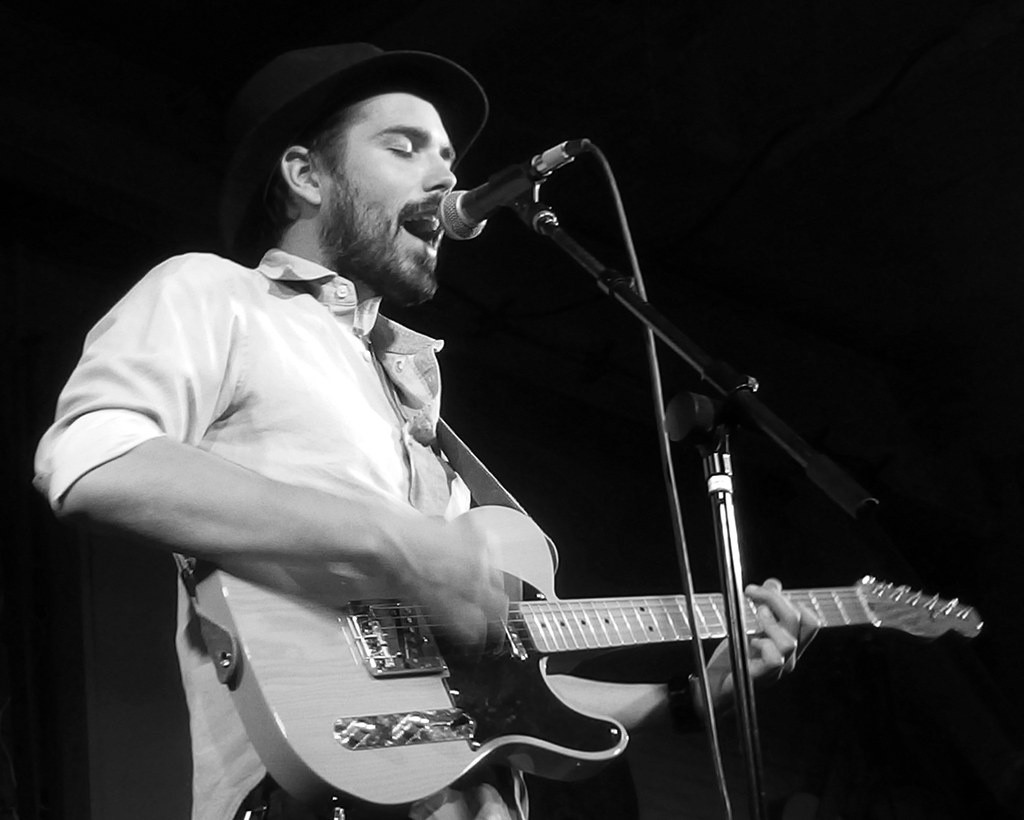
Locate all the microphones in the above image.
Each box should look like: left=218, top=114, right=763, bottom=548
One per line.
left=438, top=139, right=590, bottom=240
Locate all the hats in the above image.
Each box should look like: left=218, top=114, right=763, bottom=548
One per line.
left=220, top=42, right=490, bottom=269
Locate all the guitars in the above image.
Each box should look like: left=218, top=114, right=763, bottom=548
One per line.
left=176, top=503, right=984, bottom=820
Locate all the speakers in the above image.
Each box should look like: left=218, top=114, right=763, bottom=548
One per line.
left=34, top=521, right=194, bottom=820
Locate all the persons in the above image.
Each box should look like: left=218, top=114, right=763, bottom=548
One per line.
left=32, top=40, right=820, bottom=820
